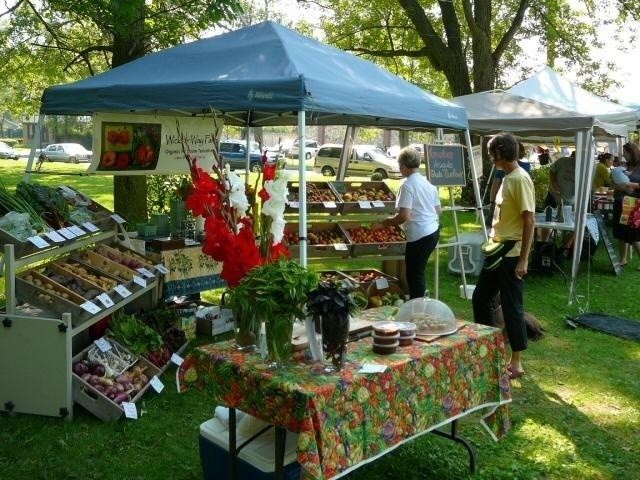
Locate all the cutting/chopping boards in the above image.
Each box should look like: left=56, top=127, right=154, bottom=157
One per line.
left=234, top=313, right=373, bottom=350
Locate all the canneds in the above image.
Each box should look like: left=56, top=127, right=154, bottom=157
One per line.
left=594, top=190, right=614, bottom=220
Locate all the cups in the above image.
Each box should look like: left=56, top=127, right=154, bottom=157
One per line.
left=460, top=285, right=476, bottom=299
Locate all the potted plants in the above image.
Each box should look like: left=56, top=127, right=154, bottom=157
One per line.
left=242, top=259, right=317, bottom=368
left=307, top=282, right=357, bottom=372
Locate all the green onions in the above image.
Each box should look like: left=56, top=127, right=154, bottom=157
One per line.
left=0, top=190, right=54, bottom=232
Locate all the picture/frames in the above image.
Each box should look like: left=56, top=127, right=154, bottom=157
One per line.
left=425, top=144, right=466, bottom=187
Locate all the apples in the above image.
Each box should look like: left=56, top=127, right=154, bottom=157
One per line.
left=343, top=187, right=394, bottom=201
left=116, top=153, right=130, bottom=169
left=101, top=151, right=117, bottom=168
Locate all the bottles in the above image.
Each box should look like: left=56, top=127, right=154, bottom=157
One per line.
left=545, top=206, right=552, bottom=221
left=555, top=191, right=564, bottom=221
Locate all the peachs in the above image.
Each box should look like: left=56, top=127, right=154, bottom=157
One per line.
left=107, top=131, right=119, bottom=143
left=119, top=132, right=128, bottom=143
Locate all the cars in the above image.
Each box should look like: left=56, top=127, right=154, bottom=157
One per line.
left=0, top=142, right=20, bottom=160
left=218, top=135, right=425, bottom=180
left=36, top=142, right=93, bottom=163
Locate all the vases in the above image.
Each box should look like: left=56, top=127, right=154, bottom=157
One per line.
left=229, top=295, right=262, bottom=350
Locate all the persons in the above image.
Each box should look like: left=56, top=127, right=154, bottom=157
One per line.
left=490, top=142, right=530, bottom=203
left=541, top=143, right=640, bottom=267
left=383, top=146, right=441, bottom=300
left=471, top=134, right=536, bottom=379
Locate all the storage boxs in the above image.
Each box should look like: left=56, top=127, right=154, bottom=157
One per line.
left=448, top=233, right=485, bottom=276
left=199, top=417, right=300, bottom=480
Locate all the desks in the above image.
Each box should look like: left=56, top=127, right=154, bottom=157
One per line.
left=177, top=306, right=512, bottom=480
left=535, top=213, right=586, bottom=289
left=118, top=231, right=231, bottom=337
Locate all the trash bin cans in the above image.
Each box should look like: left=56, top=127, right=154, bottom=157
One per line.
left=535, top=240, right=553, bottom=277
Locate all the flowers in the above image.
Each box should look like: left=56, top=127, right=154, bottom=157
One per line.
left=175, top=105, right=290, bottom=289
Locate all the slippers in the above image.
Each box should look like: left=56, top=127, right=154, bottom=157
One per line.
left=507, top=364, right=524, bottom=379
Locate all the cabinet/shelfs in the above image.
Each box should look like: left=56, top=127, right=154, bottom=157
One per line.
left=0, top=221, right=159, bottom=420
left=284, top=213, right=406, bottom=260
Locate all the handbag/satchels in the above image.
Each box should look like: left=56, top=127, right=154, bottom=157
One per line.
left=619, top=196, right=640, bottom=228
left=480, top=237, right=504, bottom=273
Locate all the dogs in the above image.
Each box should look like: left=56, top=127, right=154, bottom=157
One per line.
left=492, top=290, right=546, bottom=342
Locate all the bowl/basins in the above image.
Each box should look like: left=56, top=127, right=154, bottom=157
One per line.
left=371, top=320, right=417, bottom=352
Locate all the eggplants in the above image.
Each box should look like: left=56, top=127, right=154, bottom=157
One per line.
left=73, top=359, right=134, bottom=404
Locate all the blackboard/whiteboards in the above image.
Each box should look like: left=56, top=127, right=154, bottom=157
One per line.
left=593, top=212, right=622, bottom=275
left=424, top=143, right=467, bottom=188
